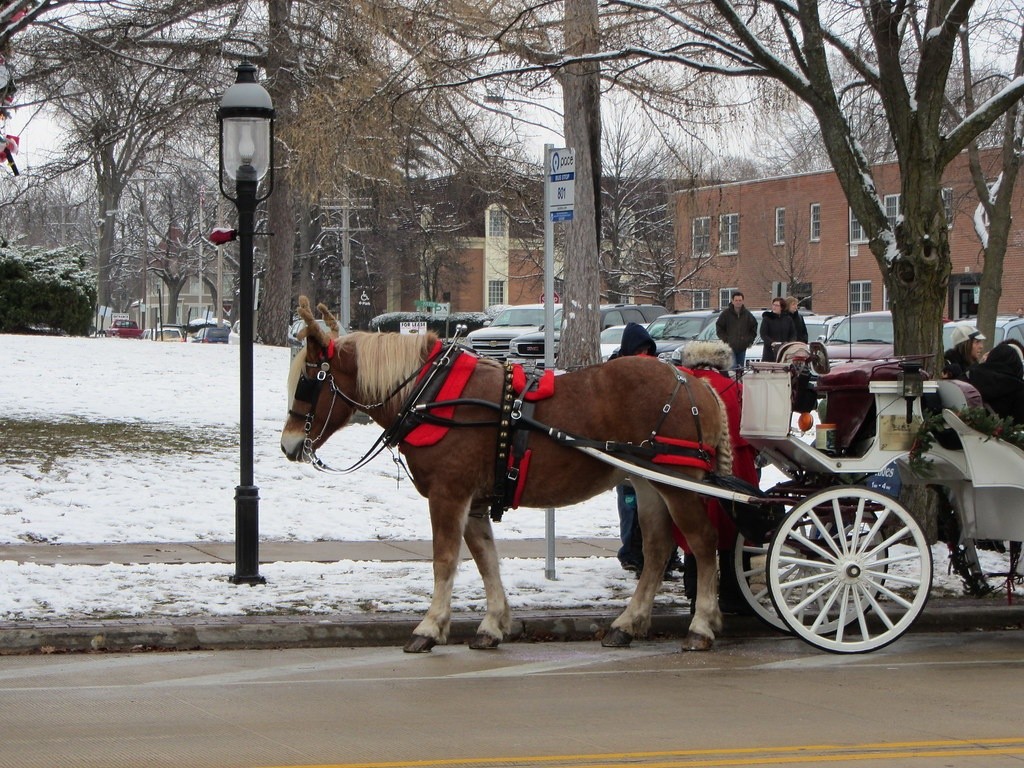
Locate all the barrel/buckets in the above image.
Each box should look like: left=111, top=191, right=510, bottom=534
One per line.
left=816, top=424, right=838, bottom=454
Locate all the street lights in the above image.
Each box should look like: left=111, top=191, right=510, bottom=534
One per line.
left=214, top=54, right=280, bottom=584
left=106, top=208, right=147, bottom=329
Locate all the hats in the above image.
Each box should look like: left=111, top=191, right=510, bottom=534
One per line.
left=682, top=340, right=736, bottom=371
left=951, top=324, right=986, bottom=346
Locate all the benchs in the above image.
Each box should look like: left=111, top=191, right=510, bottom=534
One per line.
left=931, top=377, right=987, bottom=426
left=815, top=356, right=930, bottom=392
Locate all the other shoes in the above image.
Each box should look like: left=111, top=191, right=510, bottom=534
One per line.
left=690, top=597, right=698, bottom=613
left=668, top=555, right=685, bottom=571
left=636, top=568, right=670, bottom=581
left=622, top=561, right=636, bottom=569
left=718, top=599, right=763, bottom=615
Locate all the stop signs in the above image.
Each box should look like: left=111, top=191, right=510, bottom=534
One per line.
left=540, top=291, right=560, bottom=303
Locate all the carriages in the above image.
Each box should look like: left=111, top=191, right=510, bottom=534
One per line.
left=281, top=295, right=1024, bottom=656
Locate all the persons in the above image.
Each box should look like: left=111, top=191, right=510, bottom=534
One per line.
left=943, top=325, right=989, bottom=381
left=784, top=296, right=809, bottom=345
left=976, top=338, right=1024, bottom=424
left=607, top=322, right=686, bottom=571
left=759, top=297, right=796, bottom=362
left=716, top=292, right=758, bottom=374
left=942, top=364, right=983, bottom=410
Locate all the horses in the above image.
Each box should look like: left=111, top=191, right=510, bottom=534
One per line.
left=280, top=297, right=726, bottom=652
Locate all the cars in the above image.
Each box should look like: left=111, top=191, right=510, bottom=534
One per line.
left=146, top=324, right=186, bottom=342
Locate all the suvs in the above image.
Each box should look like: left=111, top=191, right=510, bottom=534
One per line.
left=192, top=327, right=231, bottom=343
left=467, top=303, right=1024, bottom=388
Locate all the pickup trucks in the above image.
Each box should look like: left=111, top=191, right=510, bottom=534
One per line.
left=105, top=319, right=145, bottom=339
